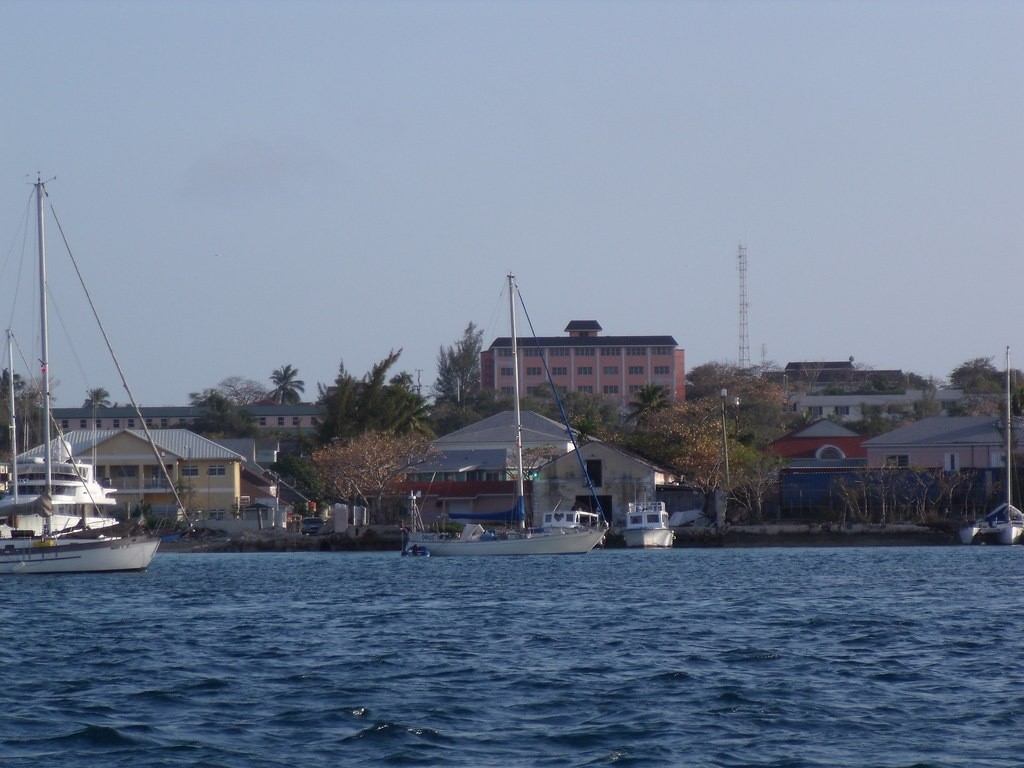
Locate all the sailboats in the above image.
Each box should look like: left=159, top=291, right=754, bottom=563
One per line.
left=0, top=169, right=196, bottom=575
left=404, top=270, right=611, bottom=555
left=913, top=345, right=1024, bottom=546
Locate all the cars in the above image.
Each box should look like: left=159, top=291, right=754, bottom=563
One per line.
left=299, top=516, right=326, bottom=535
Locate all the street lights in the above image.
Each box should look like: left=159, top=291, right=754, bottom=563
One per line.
left=718, top=388, right=742, bottom=489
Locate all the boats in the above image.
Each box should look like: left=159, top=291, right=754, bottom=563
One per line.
left=1, top=422, right=122, bottom=537
left=401, top=544, right=431, bottom=558
left=617, top=481, right=677, bottom=548
